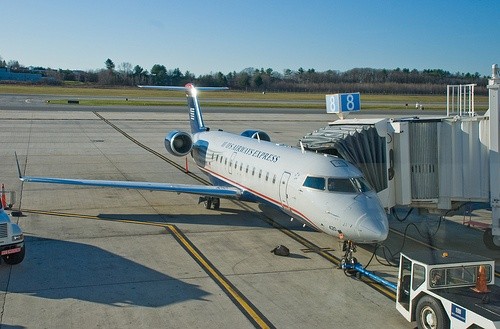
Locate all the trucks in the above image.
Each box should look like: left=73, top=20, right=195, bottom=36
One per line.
left=395, top=248, right=500, bottom=329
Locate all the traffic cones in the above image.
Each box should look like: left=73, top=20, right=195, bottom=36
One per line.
left=469, top=264, right=491, bottom=293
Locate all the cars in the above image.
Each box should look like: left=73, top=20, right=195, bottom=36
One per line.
left=0, top=190, right=26, bottom=266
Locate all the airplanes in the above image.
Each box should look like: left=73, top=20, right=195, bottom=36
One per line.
left=12, top=86, right=391, bottom=246
